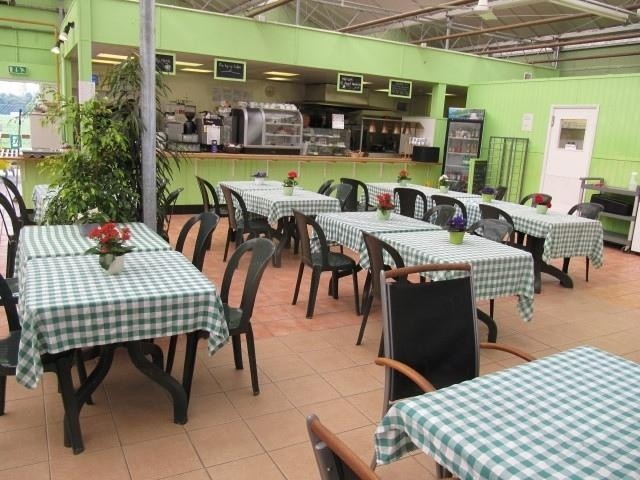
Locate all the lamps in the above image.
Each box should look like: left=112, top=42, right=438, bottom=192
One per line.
left=49, top=39, right=64, bottom=55
left=552, top=0, right=628, bottom=22
left=57, top=21, right=76, bottom=42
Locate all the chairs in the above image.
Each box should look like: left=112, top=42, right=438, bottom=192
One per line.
left=163, top=237, right=277, bottom=408
left=0, top=270, right=96, bottom=448
left=372, top=261, right=539, bottom=422
left=0, top=174, right=185, bottom=278
left=174, top=211, right=219, bottom=274
left=305, top=412, right=461, bottom=480
left=0, top=277, right=20, bottom=306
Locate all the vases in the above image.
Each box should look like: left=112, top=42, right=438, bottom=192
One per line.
left=104, top=255, right=124, bottom=275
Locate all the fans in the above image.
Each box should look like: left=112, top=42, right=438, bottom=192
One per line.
left=439, top=1, right=501, bottom=19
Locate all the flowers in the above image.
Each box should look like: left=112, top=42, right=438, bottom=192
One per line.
left=81, top=222, right=136, bottom=271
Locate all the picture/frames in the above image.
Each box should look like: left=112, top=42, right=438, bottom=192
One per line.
left=214, top=57, right=247, bottom=83
left=337, top=73, right=363, bottom=94
left=388, top=79, right=413, bottom=100
left=151, top=53, right=176, bottom=76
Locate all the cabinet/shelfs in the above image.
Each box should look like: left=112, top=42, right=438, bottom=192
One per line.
left=576, top=175, right=640, bottom=252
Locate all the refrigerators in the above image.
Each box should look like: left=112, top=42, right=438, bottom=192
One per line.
left=440, top=107, right=486, bottom=195
